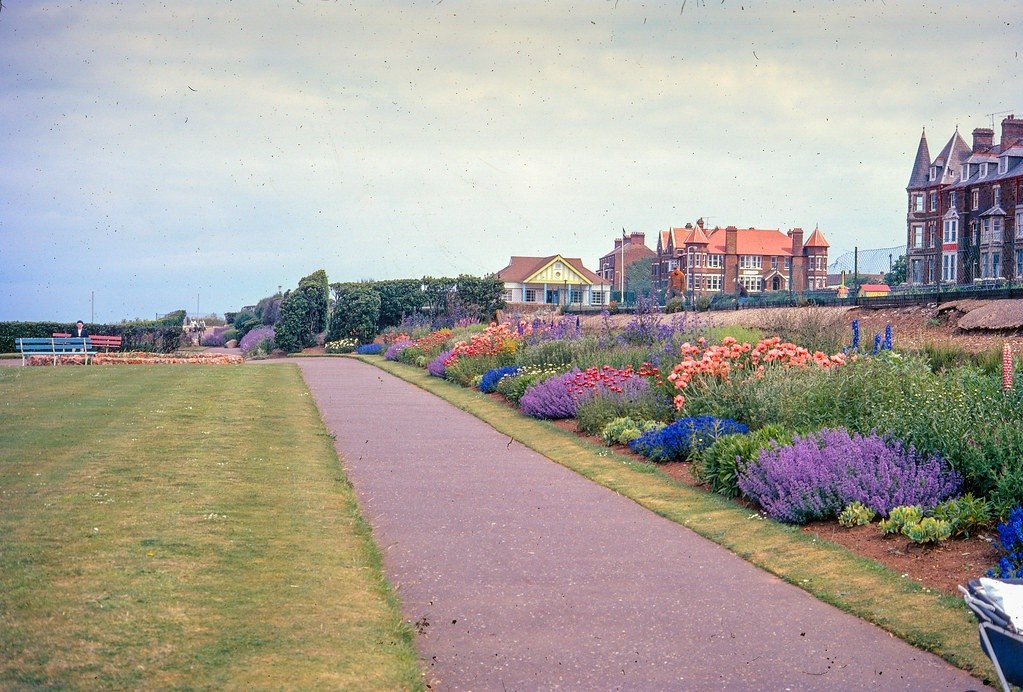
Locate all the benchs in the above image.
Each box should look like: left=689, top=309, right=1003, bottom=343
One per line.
left=15, top=338, right=98, bottom=366
left=53, top=333, right=121, bottom=353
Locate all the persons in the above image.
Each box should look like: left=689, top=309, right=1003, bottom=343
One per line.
left=666, top=266, right=686, bottom=300
left=71, top=321, right=90, bottom=352
